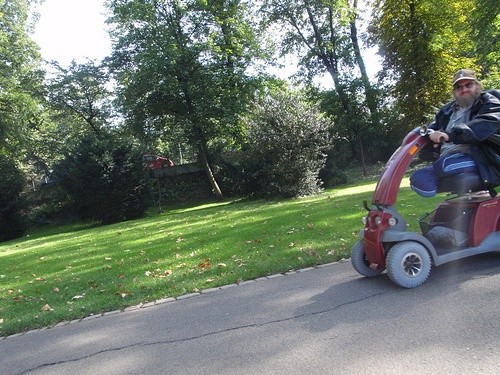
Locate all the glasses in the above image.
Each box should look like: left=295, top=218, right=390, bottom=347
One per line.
left=454, top=82, right=477, bottom=92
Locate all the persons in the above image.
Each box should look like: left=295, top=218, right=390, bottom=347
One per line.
left=411, top=69, right=500, bottom=197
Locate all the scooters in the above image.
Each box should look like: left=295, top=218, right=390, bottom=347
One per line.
left=351, top=123, right=500, bottom=288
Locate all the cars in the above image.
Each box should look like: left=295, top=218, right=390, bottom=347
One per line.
left=144, top=155, right=174, bottom=169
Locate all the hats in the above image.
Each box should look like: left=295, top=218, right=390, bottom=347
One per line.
left=452, top=69, right=481, bottom=86
